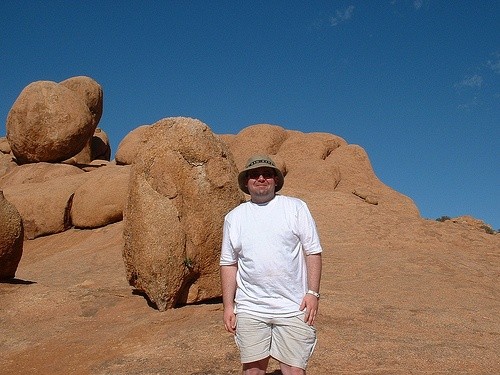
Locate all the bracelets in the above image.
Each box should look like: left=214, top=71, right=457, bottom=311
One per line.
left=307, top=290, right=320, bottom=299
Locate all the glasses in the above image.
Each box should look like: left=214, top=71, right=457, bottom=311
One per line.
left=245, top=170, right=276, bottom=180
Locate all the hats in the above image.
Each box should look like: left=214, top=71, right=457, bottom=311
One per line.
left=238, top=152, right=285, bottom=192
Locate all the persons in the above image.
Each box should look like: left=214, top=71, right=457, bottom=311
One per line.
left=219, top=154, right=323, bottom=375
